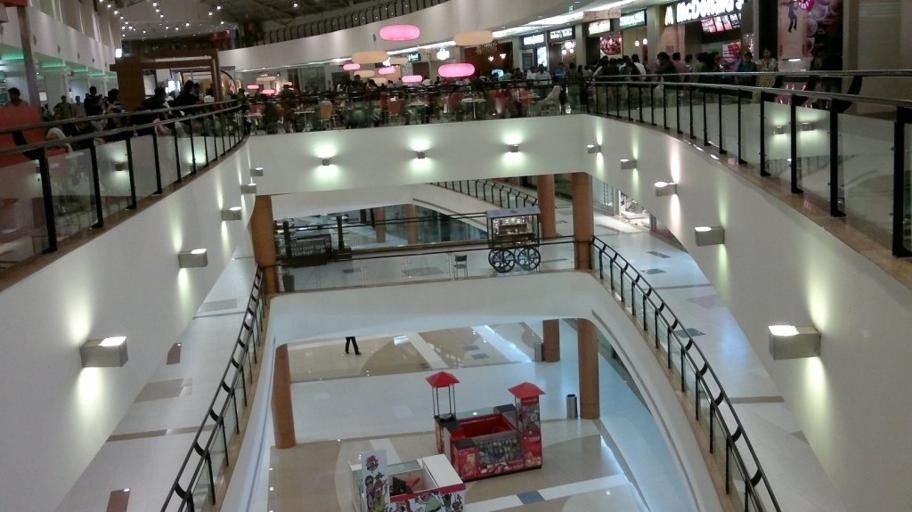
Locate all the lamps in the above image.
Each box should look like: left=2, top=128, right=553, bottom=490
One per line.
left=619, top=158, right=636, bottom=169
left=343, top=23, right=493, bottom=83
left=694, top=225, right=724, bottom=247
left=587, top=144, right=600, bottom=153
left=417, top=150, right=426, bottom=159
left=653, top=181, right=676, bottom=197
left=81, top=164, right=264, bottom=367
left=323, top=158, right=332, bottom=167
left=247, top=76, right=294, bottom=96
left=764, top=323, right=821, bottom=360
left=509, top=144, right=520, bottom=153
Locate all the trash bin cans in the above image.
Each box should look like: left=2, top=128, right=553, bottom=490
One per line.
left=283, top=272, right=296, bottom=292
left=535, top=341, right=543, bottom=361
left=567, top=394, right=577, bottom=418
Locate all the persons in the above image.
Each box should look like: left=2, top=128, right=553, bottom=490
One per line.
left=779, top=0, right=803, bottom=34
left=345, top=337, right=363, bottom=356
left=566, top=48, right=777, bottom=112
left=1, top=85, right=134, bottom=148
left=135, top=62, right=568, bottom=138
